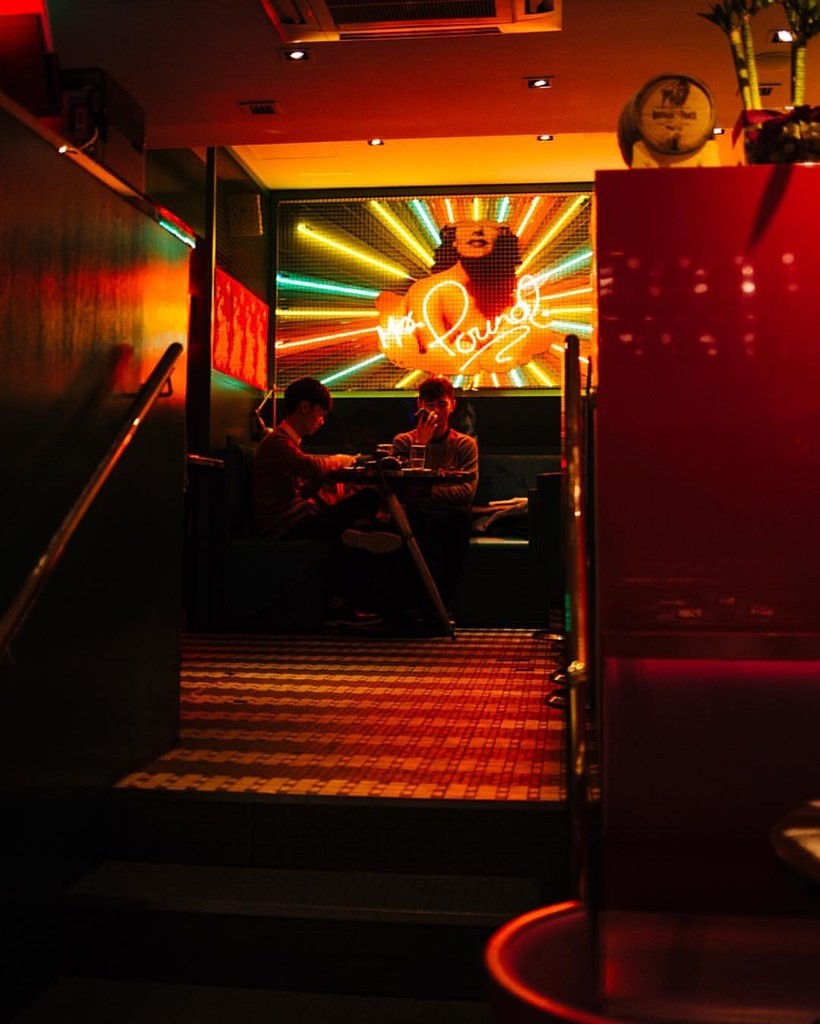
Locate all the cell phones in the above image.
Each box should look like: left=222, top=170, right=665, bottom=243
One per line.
left=414, top=408, right=439, bottom=423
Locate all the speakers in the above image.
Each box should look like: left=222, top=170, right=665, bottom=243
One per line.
left=226, top=191, right=265, bottom=237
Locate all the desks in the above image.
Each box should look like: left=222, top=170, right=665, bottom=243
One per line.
left=306, top=470, right=476, bottom=638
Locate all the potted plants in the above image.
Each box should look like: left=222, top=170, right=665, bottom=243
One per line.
left=696, top=0, right=820, bottom=162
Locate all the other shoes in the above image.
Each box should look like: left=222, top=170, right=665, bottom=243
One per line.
left=342, top=526, right=402, bottom=554
left=326, top=604, right=384, bottom=628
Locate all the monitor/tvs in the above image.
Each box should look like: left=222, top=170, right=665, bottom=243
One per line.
left=274, top=192, right=597, bottom=390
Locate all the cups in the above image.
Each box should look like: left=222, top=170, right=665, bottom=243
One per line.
left=409, top=444, right=426, bottom=469
left=376, top=442, right=395, bottom=460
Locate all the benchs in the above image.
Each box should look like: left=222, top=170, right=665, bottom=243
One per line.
left=597, top=659, right=820, bottom=1024
left=229, top=434, right=559, bottom=627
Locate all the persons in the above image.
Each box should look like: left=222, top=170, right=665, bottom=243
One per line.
left=403, top=219, right=521, bottom=362
left=247, top=376, right=404, bottom=628
left=375, top=377, right=481, bottom=628
